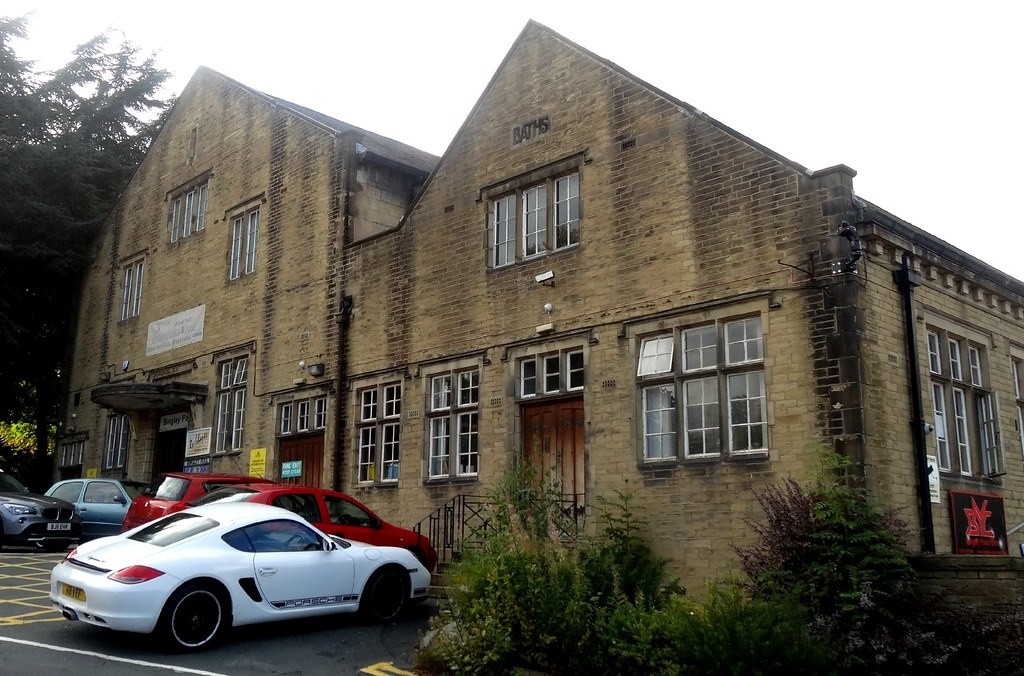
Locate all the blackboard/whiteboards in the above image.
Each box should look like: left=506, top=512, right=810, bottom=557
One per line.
left=947, top=488, right=1010, bottom=555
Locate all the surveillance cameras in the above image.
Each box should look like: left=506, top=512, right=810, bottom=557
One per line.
left=837, top=225, right=856, bottom=235
left=535, top=270, right=554, bottom=283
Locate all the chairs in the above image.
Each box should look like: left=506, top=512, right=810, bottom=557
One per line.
left=301, top=503, right=316, bottom=521
left=94, top=491, right=105, bottom=501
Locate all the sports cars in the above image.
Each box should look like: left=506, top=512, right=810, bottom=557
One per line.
left=49, top=501, right=432, bottom=651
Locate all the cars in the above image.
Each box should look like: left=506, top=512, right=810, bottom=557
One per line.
left=0, top=469, right=85, bottom=553
left=34, top=476, right=156, bottom=548
left=121, top=471, right=276, bottom=535
left=120, top=483, right=437, bottom=575
